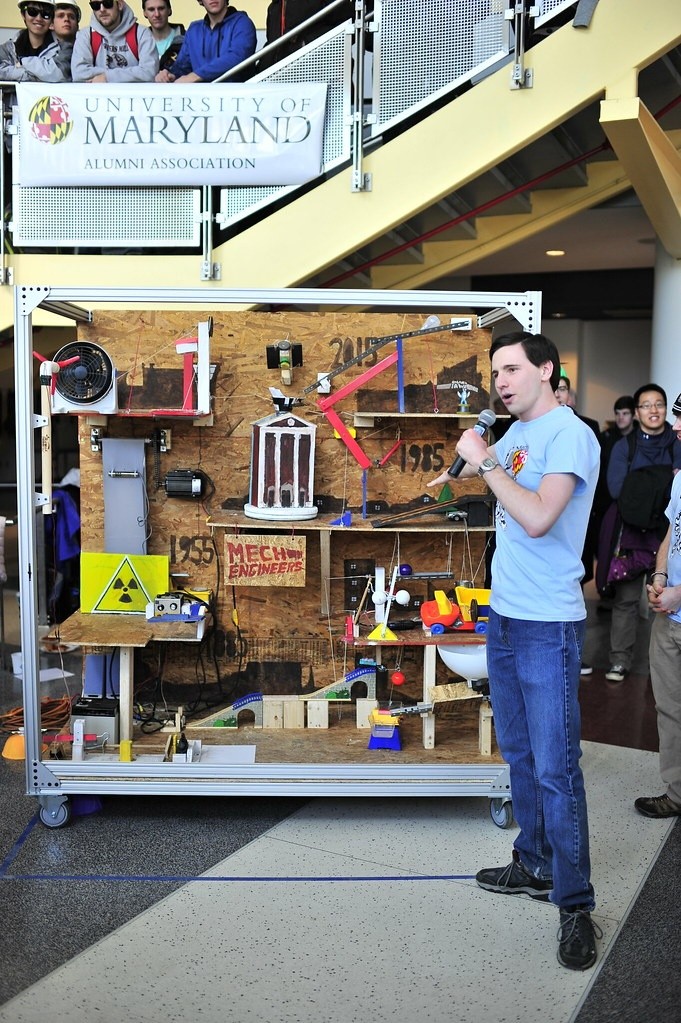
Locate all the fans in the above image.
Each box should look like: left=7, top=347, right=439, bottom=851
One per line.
left=49, top=341, right=118, bottom=414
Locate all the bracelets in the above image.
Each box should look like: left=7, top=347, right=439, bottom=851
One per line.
left=651, top=571, right=668, bottom=579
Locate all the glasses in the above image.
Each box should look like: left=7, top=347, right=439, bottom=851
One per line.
left=637, top=400, right=666, bottom=409
left=89, top=0, right=116, bottom=11
left=22, top=6, right=52, bottom=19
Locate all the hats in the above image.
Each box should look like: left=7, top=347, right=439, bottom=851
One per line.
left=672, top=393, right=681, bottom=412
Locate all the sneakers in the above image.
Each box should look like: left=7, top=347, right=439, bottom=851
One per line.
left=557, top=907, right=603, bottom=970
left=605, top=664, right=628, bottom=681
left=635, top=793, right=681, bottom=817
left=605, top=681, right=624, bottom=696
left=579, top=662, right=592, bottom=674
left=475, top=850, right=553, bottom=901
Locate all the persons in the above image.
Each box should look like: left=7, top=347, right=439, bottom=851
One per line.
left=552, top=375, right=681, bottom=819
left=0, top=0, right=472, bottom=251
left=426, top=330, right=600, bottom=972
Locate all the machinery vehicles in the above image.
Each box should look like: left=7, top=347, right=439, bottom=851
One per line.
left=420, top=586, right=493, bottom=636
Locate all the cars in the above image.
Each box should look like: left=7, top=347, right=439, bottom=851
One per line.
left=0, top=483, right=62, bottom=580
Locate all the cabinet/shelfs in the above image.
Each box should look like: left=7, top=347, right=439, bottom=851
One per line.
left=206, top=412, right=513, bottom=618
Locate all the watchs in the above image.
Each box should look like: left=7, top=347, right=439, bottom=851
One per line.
left=478, top=457, right=500, bottom=477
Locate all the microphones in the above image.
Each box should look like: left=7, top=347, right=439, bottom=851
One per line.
left=448, top=409, right=497, bottom=479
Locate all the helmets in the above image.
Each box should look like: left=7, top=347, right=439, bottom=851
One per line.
left=53, top=0, right=81, bottom=21
left=17, top=0, right=57, bottom=9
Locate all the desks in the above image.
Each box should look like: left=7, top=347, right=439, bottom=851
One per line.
left=354, top=626, right=488, bottom=749
left=48, top=601, right=209, bottom=762
